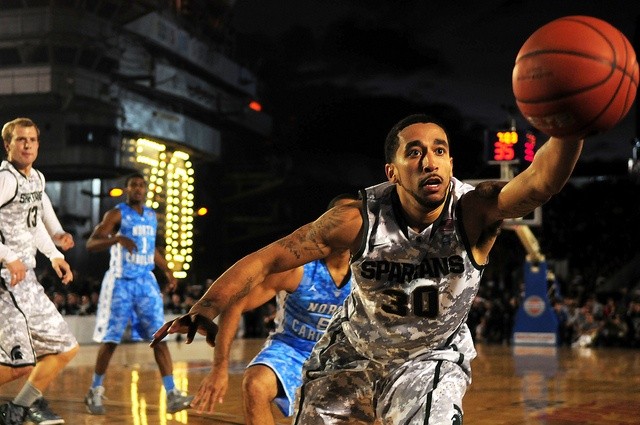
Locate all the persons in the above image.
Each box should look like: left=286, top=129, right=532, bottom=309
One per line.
left=150, top=114, right=584, bottom=424
left=190, top=194, right=363, bottom=425
left=25, top=191, right=80, bottom=425
left=0, top=117, right=73, bottom=425
left=163, top=278, right=214, bottom=314
left=85, top=175, right=194, bottom=414
left=237, top=302, right=280, bottom=339
left=45, top=290, right=98, bottom=315
left=474, top=263, right=639, bottom=347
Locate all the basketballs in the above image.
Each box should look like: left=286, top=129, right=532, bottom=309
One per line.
left=511, top=15, right=640, bottom=139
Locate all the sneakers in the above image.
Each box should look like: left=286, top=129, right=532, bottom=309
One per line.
left=83, top=385, right=108, bottom=415
left=166, top=390, right=194, bottom=415
left=0, top=399, right=26, bottom=425
left=27, top=398, right=65, bottom=424
